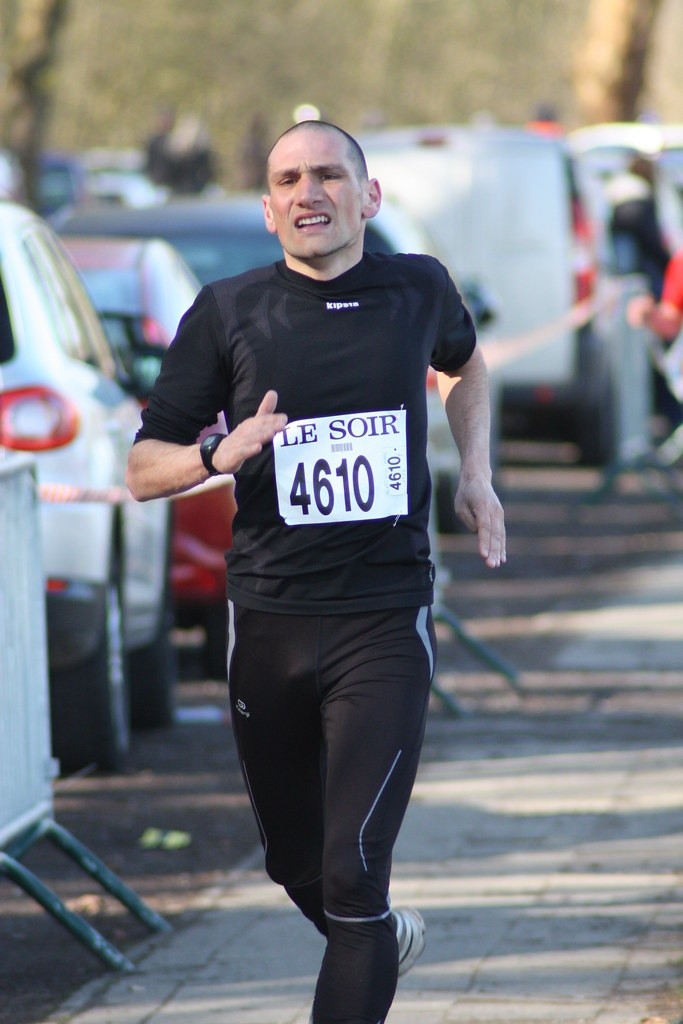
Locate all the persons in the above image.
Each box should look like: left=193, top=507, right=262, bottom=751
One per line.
left=125, top=120, right=507, bottom=1024
left=611, top=163, right=682, bottom=471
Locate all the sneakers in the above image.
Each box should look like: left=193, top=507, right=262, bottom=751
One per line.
left=391, top=906, right=426, bottom=976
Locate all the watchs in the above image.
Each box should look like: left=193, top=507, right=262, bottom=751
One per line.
left=200, top=433, right=228, bottom=476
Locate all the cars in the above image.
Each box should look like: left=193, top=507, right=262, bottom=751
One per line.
left=0, top=121, right=683, bottom=477
left=0, top=200, right=195, bottom=770
left=57, top=238, right=244, bottom=675
